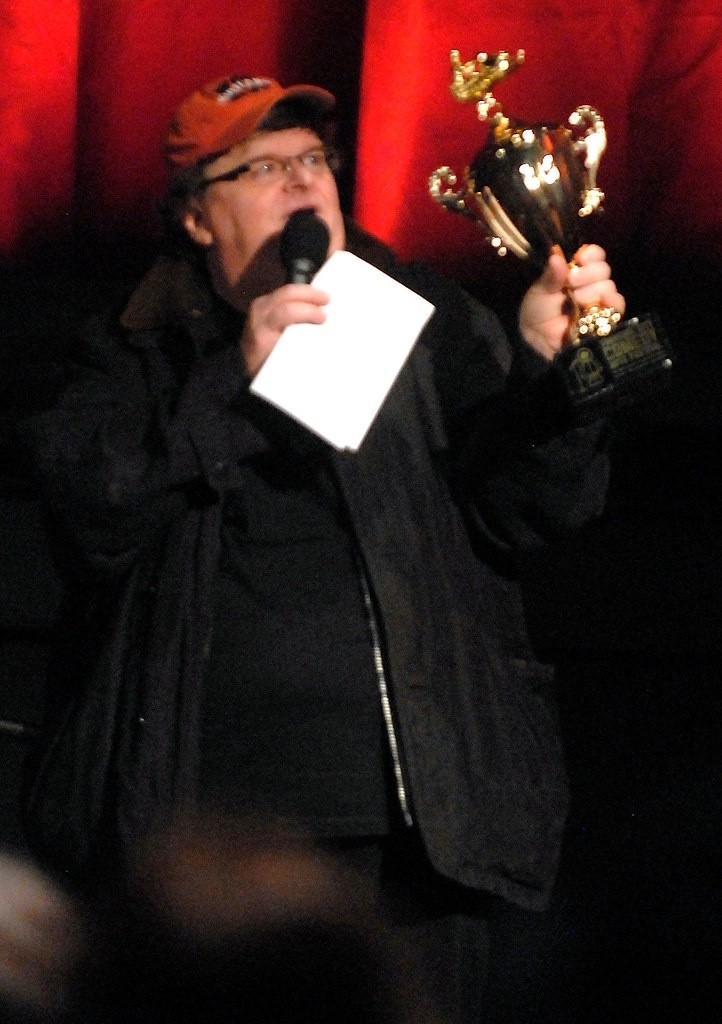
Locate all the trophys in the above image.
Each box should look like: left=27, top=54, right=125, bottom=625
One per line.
left=426, top=46, right=679, bottom=412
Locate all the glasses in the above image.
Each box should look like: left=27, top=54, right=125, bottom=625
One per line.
left=192, top=143, right=341, bottom=191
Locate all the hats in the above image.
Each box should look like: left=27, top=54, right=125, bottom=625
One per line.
left=164, top=71, right=336, bottom=180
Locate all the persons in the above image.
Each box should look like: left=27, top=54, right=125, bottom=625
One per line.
left=2, top=64, right=630, bottom=1023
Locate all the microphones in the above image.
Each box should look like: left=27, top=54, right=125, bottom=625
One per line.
left=279, top=213, right=329, bottom=286
